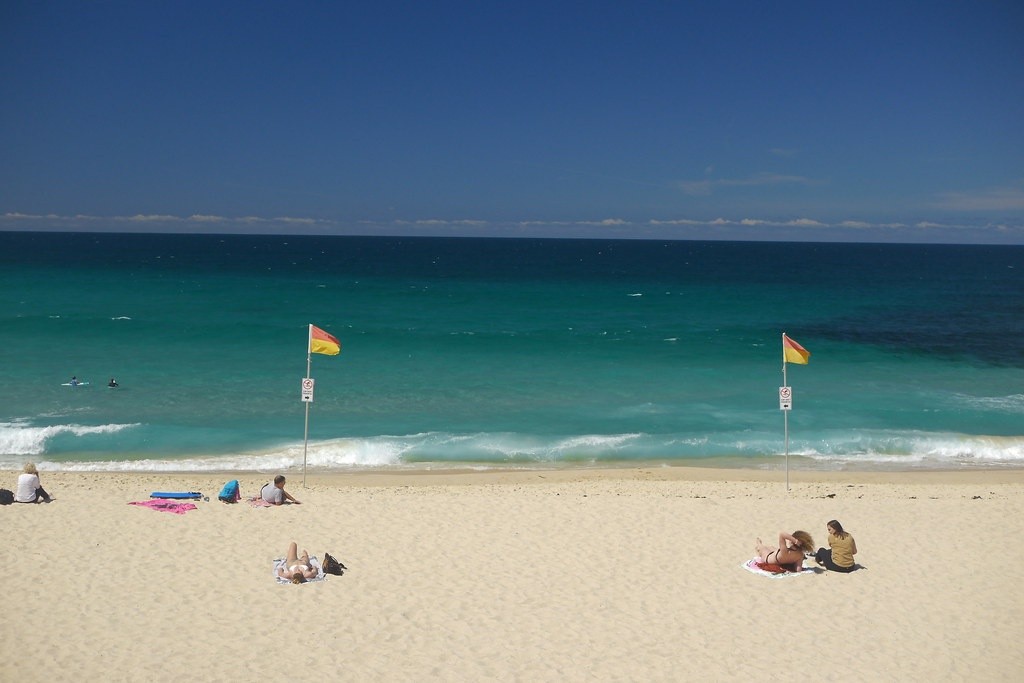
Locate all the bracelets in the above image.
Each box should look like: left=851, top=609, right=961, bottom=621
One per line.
left=795, top=540, right=798, bottom=544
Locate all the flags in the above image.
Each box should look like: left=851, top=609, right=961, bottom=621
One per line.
left=310, top=325, right=340, bottom=356
left=784, top=335, right=810, bottom=365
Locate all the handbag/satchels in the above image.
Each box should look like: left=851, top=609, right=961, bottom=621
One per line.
left=0, top=489, right=14, bottom=505
left=322, top=553, right=348, bottom=576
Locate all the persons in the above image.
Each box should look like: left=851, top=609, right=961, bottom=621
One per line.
left=17, top=463, right=52, bottom=503
left=813, top=520, right=857, bottom=573
left=260, top=475, right=300, bottom=505
left=71, top=376, right=77, bottom=384
left=755, top=530, right=815, bottom=571
left=109, top=379, right=118, bottom=387
left=278, top=542, right=317, bottom=584
